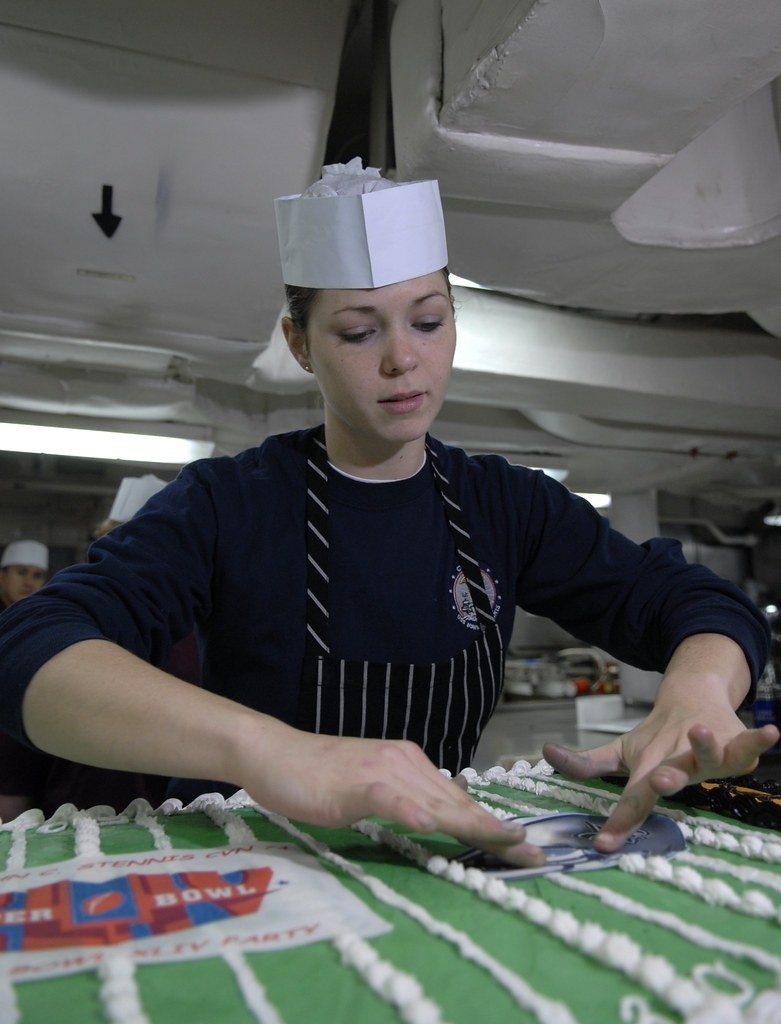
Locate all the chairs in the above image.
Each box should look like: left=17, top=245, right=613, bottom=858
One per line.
left=575, top=695, right=625, bottom=755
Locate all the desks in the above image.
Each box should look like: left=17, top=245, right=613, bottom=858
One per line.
left=578, top=719, right=639, bottom=734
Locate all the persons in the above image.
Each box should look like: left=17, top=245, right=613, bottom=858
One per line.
left=0, top=155, right=781, bottom=865
left=0, top=539, right=50, bottom=614
left=74, top=473, right=169, bottom=563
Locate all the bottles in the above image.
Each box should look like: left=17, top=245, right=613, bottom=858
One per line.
left=752, top=605, right=781, bottom=758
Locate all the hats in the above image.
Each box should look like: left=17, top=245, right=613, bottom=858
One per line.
left=274, top=157, right=449, bottom=289
left=0, top=540, right=50, bottom=571
left=111, top=474, right=169, bottom=523
